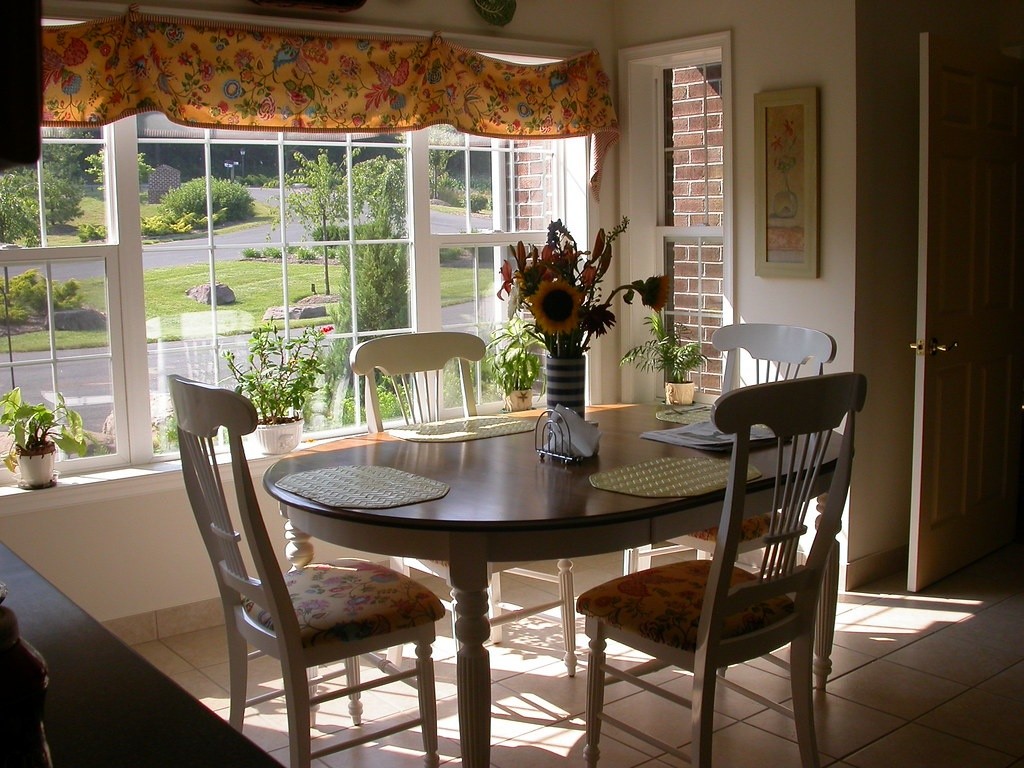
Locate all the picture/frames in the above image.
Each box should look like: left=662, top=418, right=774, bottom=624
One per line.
left=752, top=87, right=818, bottom=279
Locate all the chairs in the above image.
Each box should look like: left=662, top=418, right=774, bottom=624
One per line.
left=625, top=321, right=838, bottom=574
left=348, top=329, right=580, bottom=678
left=164, top=373, right=446, bottom=768
left=577, top=372, right=866, bottom=768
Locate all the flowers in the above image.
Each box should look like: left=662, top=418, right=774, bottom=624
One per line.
left=223, top=316, right=333, bottom=421
left=494, top=215, right=672, bottom=362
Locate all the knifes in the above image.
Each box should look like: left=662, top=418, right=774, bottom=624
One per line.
left=664, top=405, right=708, bottom=415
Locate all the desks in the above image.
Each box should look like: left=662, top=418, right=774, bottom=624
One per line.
left=258, top=404, right=854, bottom=768
left=0, top=540, right=284, bottom=768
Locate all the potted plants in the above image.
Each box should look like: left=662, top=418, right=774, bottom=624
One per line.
left=482, top=317, right=547, bottom=411
left=627, top=316, right=710, bottom=406
left=0, top=385, right=98, bottom=491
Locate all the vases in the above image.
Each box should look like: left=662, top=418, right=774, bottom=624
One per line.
left=544, top=356, right=586, bottom=429
left=255, top=419, right=303, bottom=456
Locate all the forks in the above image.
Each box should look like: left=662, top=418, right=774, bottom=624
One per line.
left=671, top=408, right=710, bottom=414
